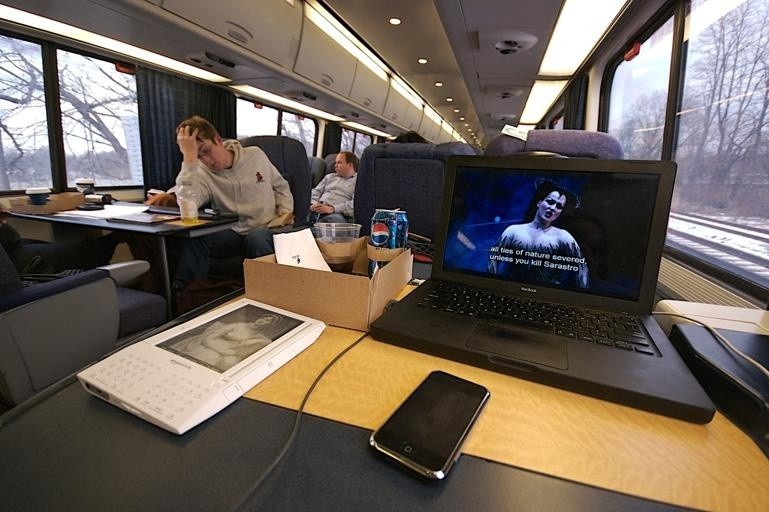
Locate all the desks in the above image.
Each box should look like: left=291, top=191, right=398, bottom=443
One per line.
left=0, top=262, right=768, bottom=512
left=4, top=201, right=239, bottom=320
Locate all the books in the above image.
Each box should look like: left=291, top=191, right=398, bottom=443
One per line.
left=106, top=212, right=181, bottom=226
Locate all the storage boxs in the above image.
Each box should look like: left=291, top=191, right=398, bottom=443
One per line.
left=244, top=240, right=413, bottom=334
left=6, top=192, right=84, bottom=214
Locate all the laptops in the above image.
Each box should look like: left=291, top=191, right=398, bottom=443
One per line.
left=370, top=155, right=718, bottom=425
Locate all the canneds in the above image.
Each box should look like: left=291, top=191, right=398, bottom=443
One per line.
left=367, top=208, right=409, bottom=280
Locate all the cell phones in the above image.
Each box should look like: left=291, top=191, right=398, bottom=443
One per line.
left=368, top=368, right=490, bottom=485
left=204, top=208, right=224, bottom=216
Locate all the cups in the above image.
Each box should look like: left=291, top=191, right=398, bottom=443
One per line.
left=312, top=221, right=364, bottom=265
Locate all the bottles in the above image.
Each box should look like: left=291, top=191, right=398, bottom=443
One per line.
left=24, top=187, right=53, bottom=206
left=74, top=178, right=97, bottom=198
left=176, top=175, right=200, bottom=229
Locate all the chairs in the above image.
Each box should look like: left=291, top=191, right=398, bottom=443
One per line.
left=483, top=128, right=628, bottom=158
left=351, top=140, right=480, bottom=279
left=0, top=245, right=167, bottom=407
left=307, top=157, right=327, bottom=189
left=324, top=154, right=336, bottom=174
left=210, top=136, right=311, bottom=290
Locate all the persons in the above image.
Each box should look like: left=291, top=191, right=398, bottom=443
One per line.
left=185, top=314, right=283, bottom=369
left=141, top=113, right=358, bottom=290
left=489, top=177, right=591, bottom=291
